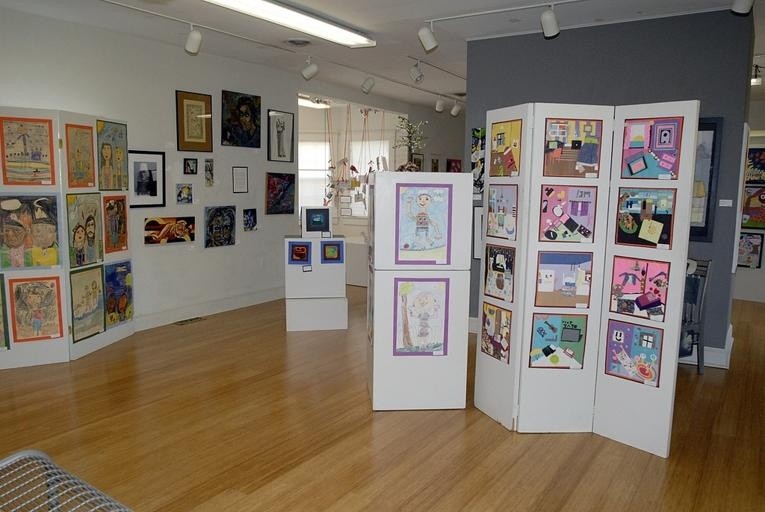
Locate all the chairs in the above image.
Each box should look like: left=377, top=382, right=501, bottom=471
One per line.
left=679, top=258, right=713, bottom=376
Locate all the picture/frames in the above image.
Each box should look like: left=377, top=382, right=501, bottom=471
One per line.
left=410, top=153, right=424, bottom=173
left=288, top=238, right=312, bottom=267
left=319, top=240, right=345, bottom=265
left=690, top=117, right=724, bottom=243
left=183, top=156, right=198, bottom=175
left=128, top=149, right=166, bottom=209
left=737, top=231, right=764, bottom=268
left=303, top=207, right=332, bottom=234
left=267, top=108, right=295, bottom=163
left=173, top=88, right=213, bottom=153
left=744, top=146, right=765, bottom=186
left=740, top=185, right=765, bottom=230
left=231, top=165, right=249, bottom=193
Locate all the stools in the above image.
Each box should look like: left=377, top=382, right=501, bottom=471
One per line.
left=0, top=446, right=137, bottom=511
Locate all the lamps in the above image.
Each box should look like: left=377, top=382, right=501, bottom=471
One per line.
left=418, top=0, right=755, bottom=52
left=203, top=0, right=378, bottom=50
left=749, top=57, right=764, bottom=89
left=183, top=20, right=463, bottom=119
left=404, top=56, right=467, bottom=87
